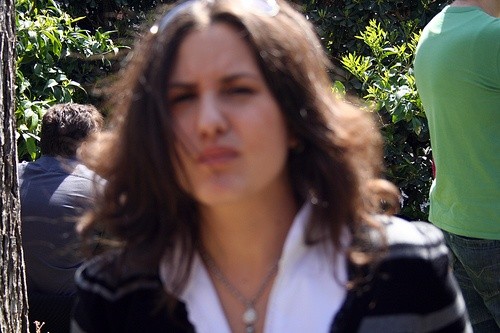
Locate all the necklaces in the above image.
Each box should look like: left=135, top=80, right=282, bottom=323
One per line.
left=205, top=254, right=279, bottom=333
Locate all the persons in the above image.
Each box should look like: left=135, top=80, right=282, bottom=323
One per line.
left=72, top=0, right=473, bottom=333
left=16, top=102, right=111, bottom=333
left=413, top=0, right=500, bottom=333
